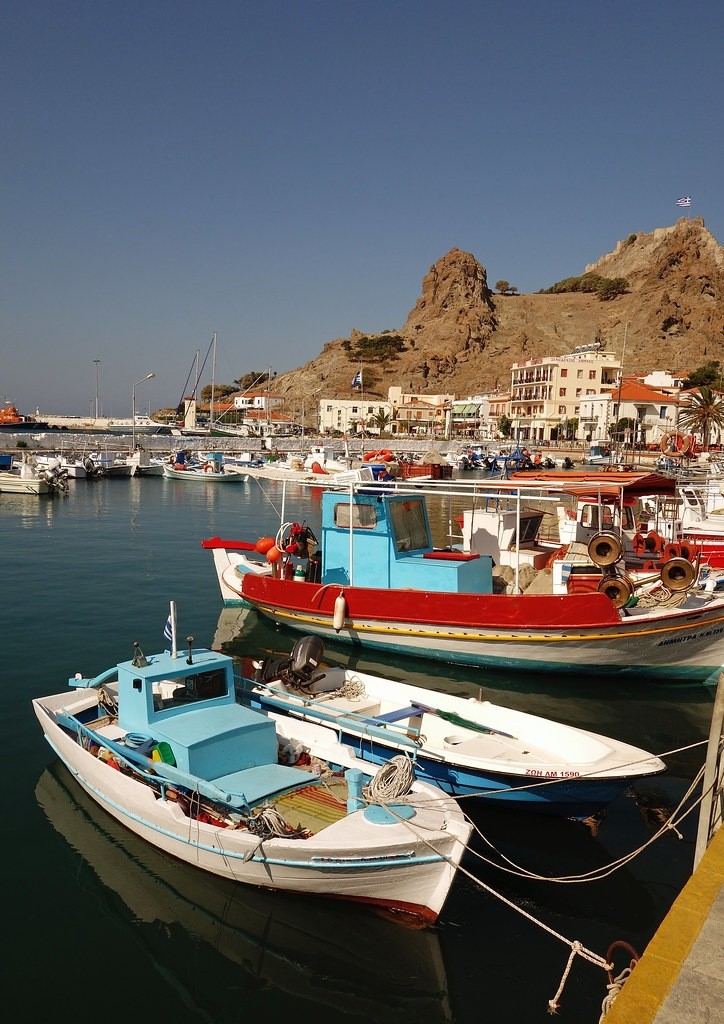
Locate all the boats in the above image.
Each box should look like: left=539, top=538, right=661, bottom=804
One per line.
left=0, top=321, right=723, bottom=596
left=200, top=464, right=724, bottom=684
left=34, top=756, right=457, bottom=1024
left=30, top=599, right=476, bottom=931
left=207, top=602, right=669, bottom=824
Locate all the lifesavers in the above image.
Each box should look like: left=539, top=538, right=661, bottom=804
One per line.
left=363, top=450, right=394, bottom=463
left=204, top=464, right=214, bottom=473
left=661, top=430, right=692, bottom=458
left=467, top=448, right=473, bottom=455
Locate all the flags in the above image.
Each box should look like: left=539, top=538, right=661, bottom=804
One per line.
left=351, top=370, right=361, bottom=389
left=163, top=613, right=172, bottom=641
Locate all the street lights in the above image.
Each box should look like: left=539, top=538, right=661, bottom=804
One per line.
left=93, top=360, right=101, bottom=419
left=132, top=373, right=155, bottom=454
